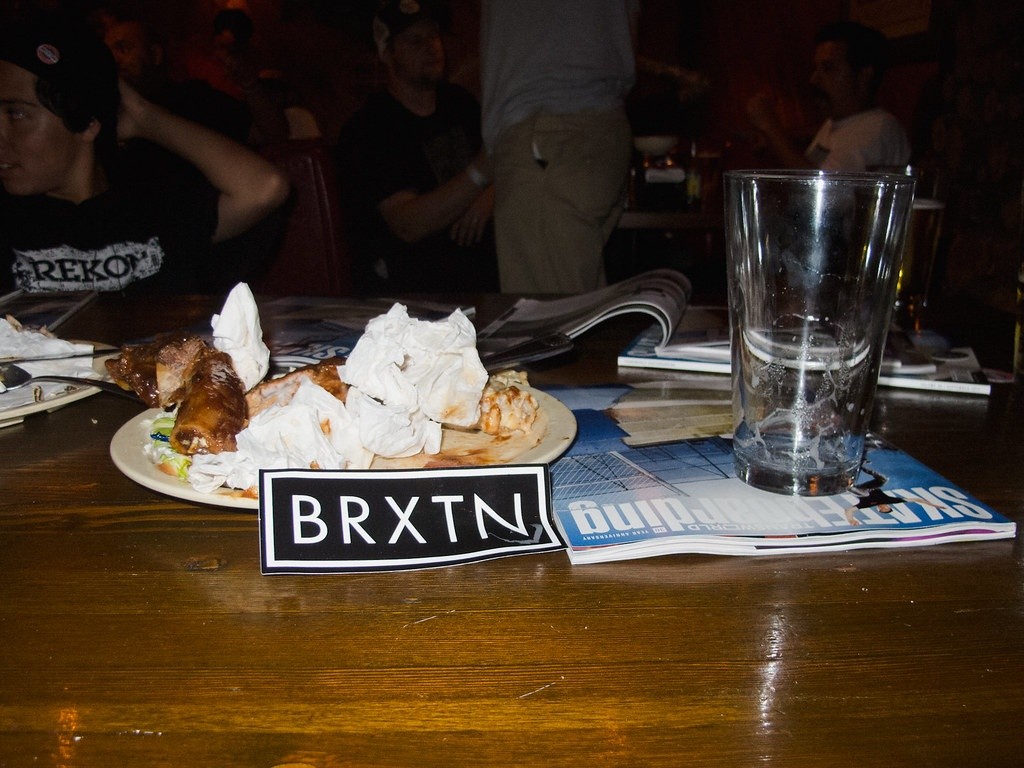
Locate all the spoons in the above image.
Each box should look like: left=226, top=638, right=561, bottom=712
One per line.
left=0, top=362, right=146, bottom=403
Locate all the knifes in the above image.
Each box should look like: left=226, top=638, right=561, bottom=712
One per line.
left=0, top=349, right=120, bottom=363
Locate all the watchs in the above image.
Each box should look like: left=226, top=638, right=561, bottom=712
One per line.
left=467, top=165, right=491, bottom=189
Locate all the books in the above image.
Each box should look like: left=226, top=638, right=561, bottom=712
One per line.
left=468, top=269, right=991, bottom=396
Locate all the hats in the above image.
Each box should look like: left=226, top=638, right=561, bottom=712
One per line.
left=0, top=14, right=118, bottom=137
left=373, top=0, right=433, bottom=51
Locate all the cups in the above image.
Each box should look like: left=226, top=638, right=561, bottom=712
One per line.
left=723, top=170, right=915, bottom=496
left=866, top=166, right=947, bottom=332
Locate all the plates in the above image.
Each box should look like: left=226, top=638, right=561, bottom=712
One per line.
left=0, top=339, right=122, bottom=426
left=110, top=378, right=576, bottom=509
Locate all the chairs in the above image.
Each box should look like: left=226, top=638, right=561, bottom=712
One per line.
left=220, top=135, right=355, bottom=299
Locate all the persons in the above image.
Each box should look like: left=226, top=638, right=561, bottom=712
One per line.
left=101, top=6, right=253, bottom=283
left=321, top=0, right=501, bottom=304
left=0, top=31, right=283, bottom=343
left=481, top=0, right=635, bottom=297
left=743, top=25, right=911, bottom=175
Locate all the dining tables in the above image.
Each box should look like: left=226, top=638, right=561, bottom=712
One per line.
left=0, top=294, right=1024, bottom=768
